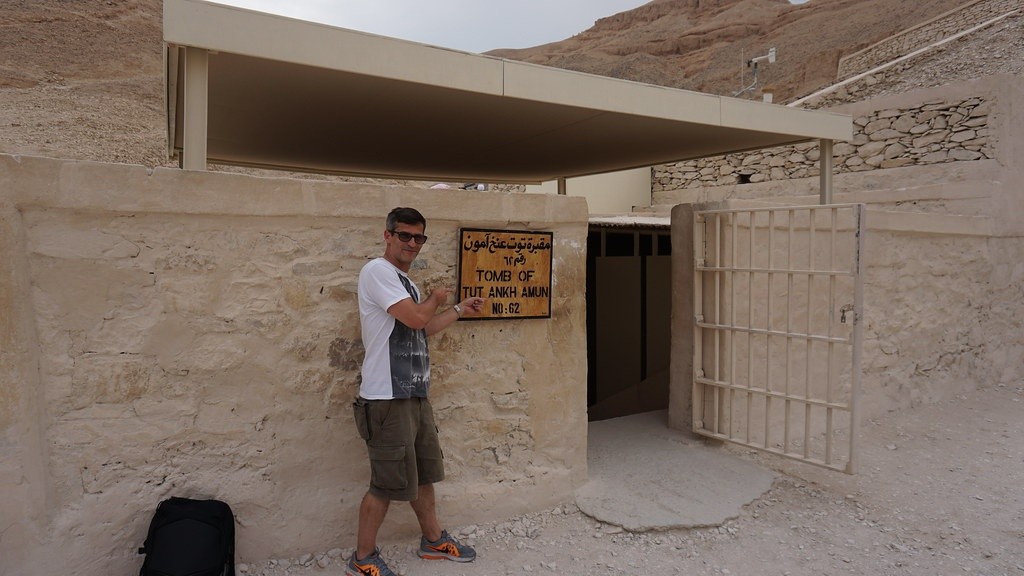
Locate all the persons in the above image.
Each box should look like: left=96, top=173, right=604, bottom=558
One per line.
left=348, top=207, right=486, bottom=576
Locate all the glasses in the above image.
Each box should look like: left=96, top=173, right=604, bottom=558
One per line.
left=394, top=230, right=427, bottom=244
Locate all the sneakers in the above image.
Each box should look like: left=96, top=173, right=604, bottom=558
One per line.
left=346, top=546, right=396, bottom=576
left=417, top=529, right=476, bottom=562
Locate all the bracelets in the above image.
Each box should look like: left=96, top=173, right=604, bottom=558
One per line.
left=453, top=305, right=462, bottom=318
